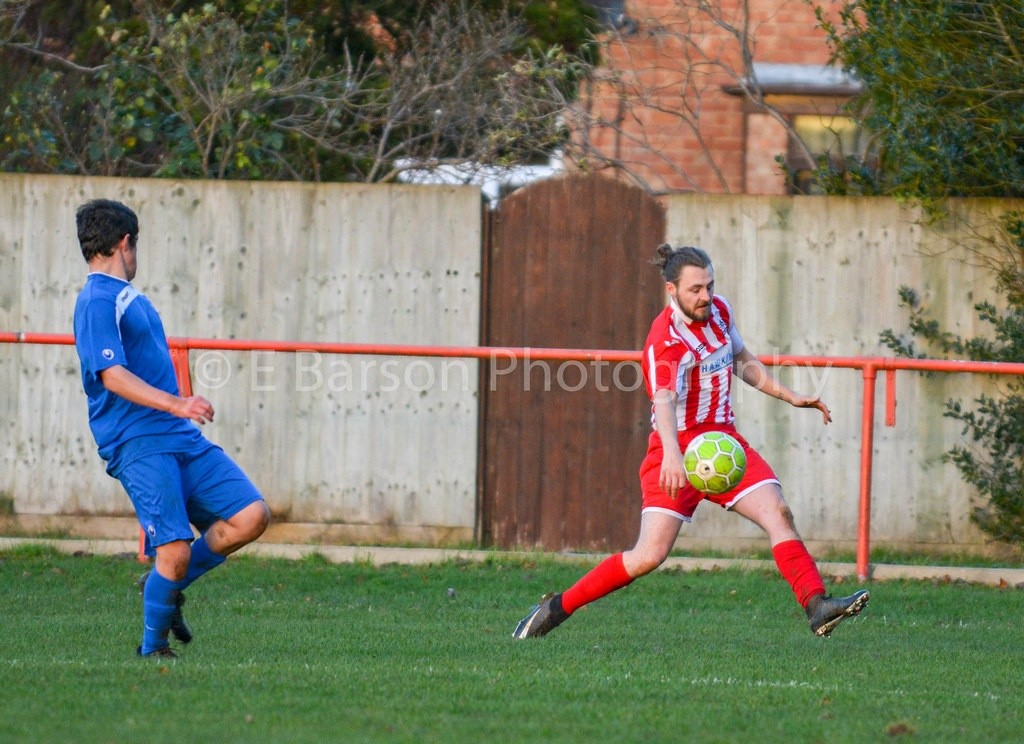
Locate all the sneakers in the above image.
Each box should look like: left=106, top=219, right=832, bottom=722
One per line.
left=136, top=646, right=175, bottom=660
left=139, top=571, right=193, bottom=643
left=513, top=591, right=561, bottom=640
left=806, top=589, right=870, bottom=635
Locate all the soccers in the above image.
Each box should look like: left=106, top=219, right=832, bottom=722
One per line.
left=680, top=427, right=752, bottom=498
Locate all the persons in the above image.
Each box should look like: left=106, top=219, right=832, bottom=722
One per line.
left=511, top=245, right=871, bottom=641
left=76, top=199, right=271, bottom=657
